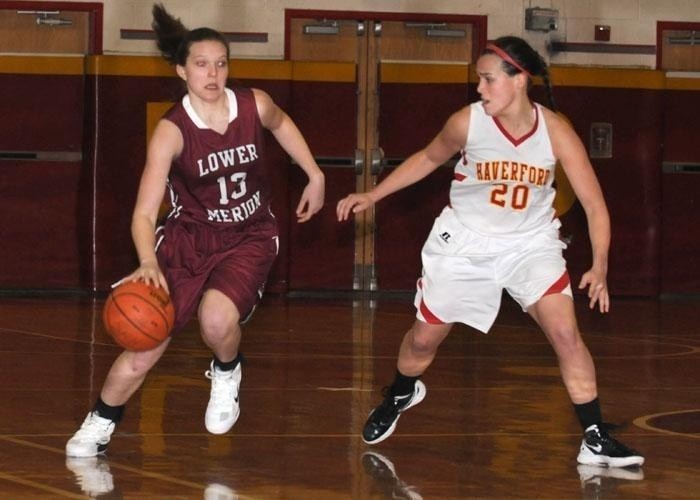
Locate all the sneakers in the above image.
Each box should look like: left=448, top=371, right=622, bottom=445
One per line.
left=360, top=376, right=428, bottom=445
left=205, top=483, right=238, bottom=500
left=65, top=409, right=117, bottom=458
left=361, top=452, right=422, bottom=499
left=576, top=465, right=645, bottom=495
left=204, top=360, right=243, bottom=435
left=576, top=429, right=645, bottom=469
left=65, top=455, right=115, bottom=496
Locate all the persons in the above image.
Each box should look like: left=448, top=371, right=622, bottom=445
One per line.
left=337, top=36, right=645, bottom=469
left=360, top=453, right=645, bottom=500
left=64, top=4, right=325, bottom=459
left=65, top=460, right=238, bottom=500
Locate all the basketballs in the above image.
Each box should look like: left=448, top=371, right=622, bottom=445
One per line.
left=102, top=279, right=175, bottom=352
